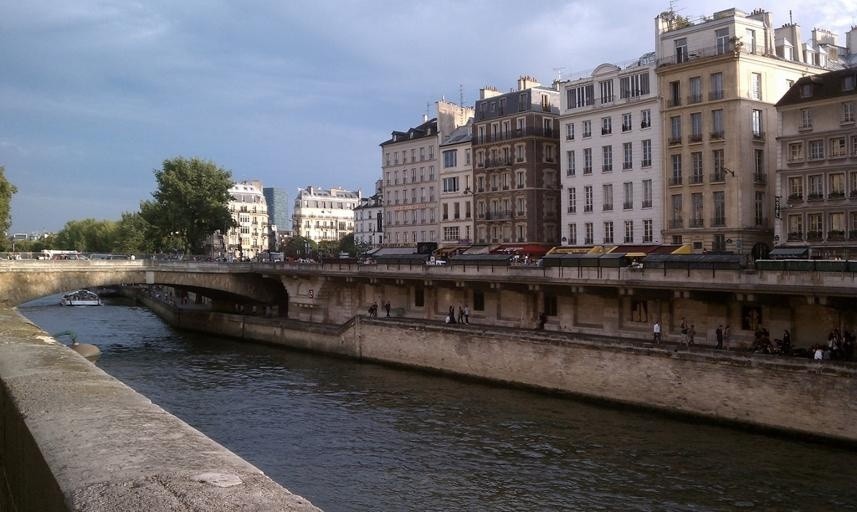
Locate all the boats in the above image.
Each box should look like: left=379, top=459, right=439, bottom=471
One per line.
left=58, top=289, right=104, bottom=307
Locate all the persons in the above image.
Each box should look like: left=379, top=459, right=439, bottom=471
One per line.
left=120, top=283, right=188, bottom=305
left=812, top=328, right=857, bottom=360
left=652, top=316, right=696, bottom=348
left=714, top=324, right=723, bottom=349
left=447, top=303, right=471, bottom=326
left=385, top=300, right=390, bottom=316
left=754, top=323, right=791, bottom=355
left=510, top=256, right=533, bottom=264
left=835, top=255, right=856, bottom=260
left=539, top=311, right=548, bottom=330
left=368, top=304, right=377, bottom=317
left=127, top=253, right=313, bottom=263
left=373, top=302, right=377, bottom=317
left=5, top=250, right=70, bottom=261
left=65, top=291, right=97, bottom=301
left=724, top=324, right=732, bottom=351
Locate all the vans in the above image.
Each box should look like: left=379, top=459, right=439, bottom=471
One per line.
left=41, top=250, right=89, bottom=261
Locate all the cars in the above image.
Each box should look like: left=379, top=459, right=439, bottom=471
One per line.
left=426, top=256, right=447, bottom=267
left=89, top=249, right=318, bottom=265
left=357, top=257, right=377, bottom=265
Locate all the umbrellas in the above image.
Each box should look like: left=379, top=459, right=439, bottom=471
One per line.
left=523, top=244, right=546, bottom=264
